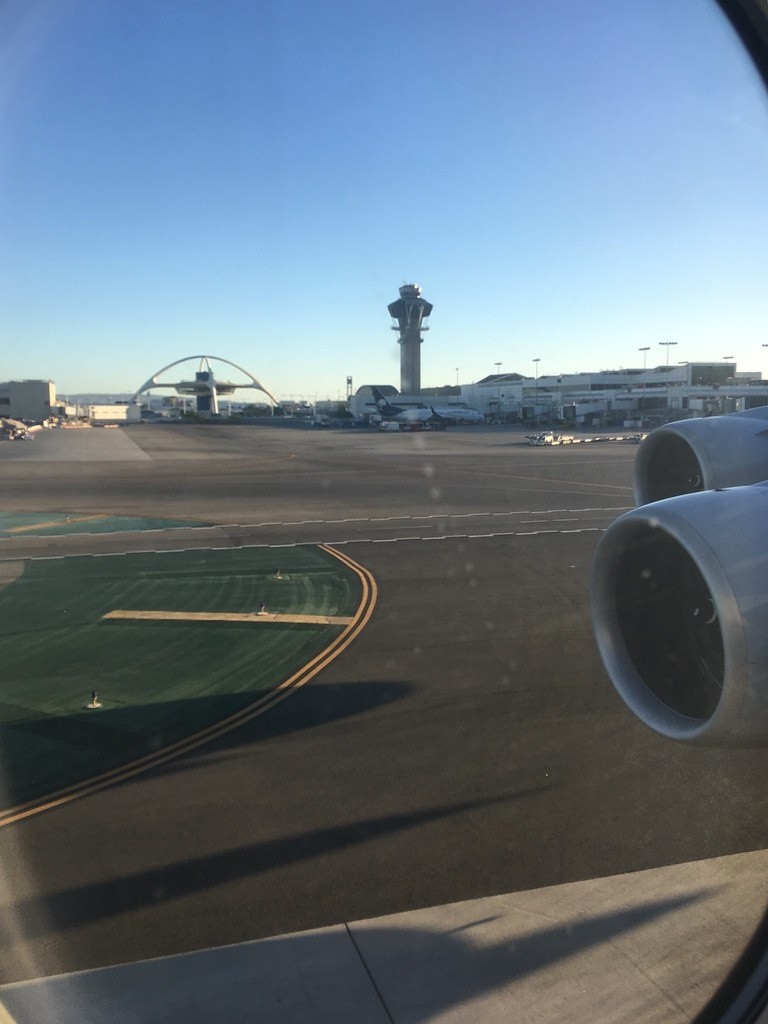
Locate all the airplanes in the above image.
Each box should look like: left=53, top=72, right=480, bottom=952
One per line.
left=365, top=386, right=484, bottom=430
